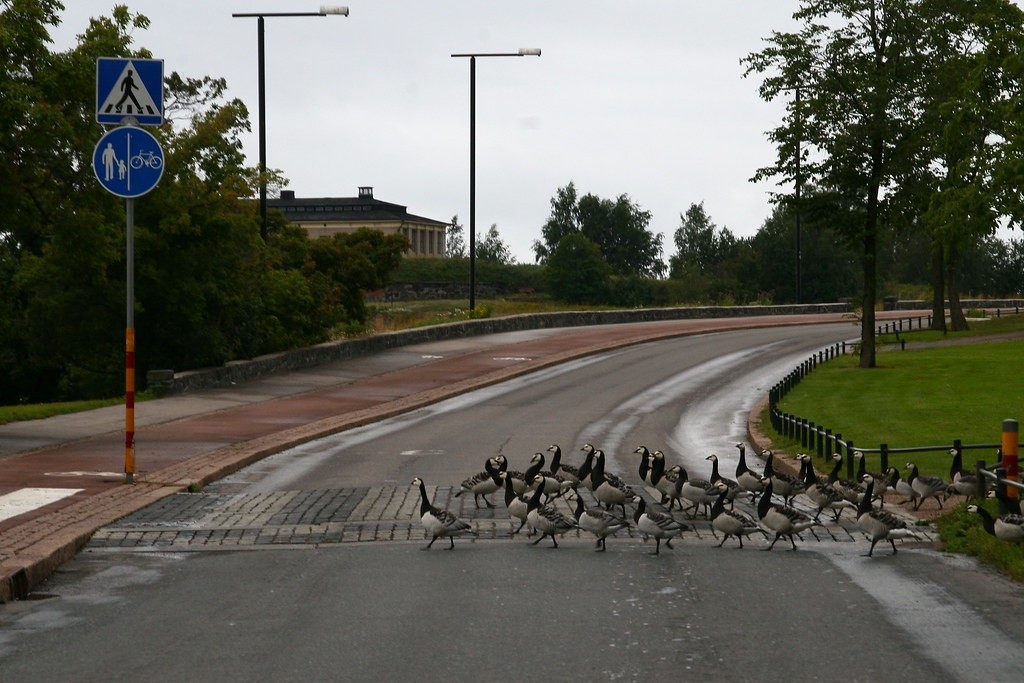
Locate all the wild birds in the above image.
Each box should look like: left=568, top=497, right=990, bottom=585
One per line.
left=410, top=443, right=1024, bottom=557
